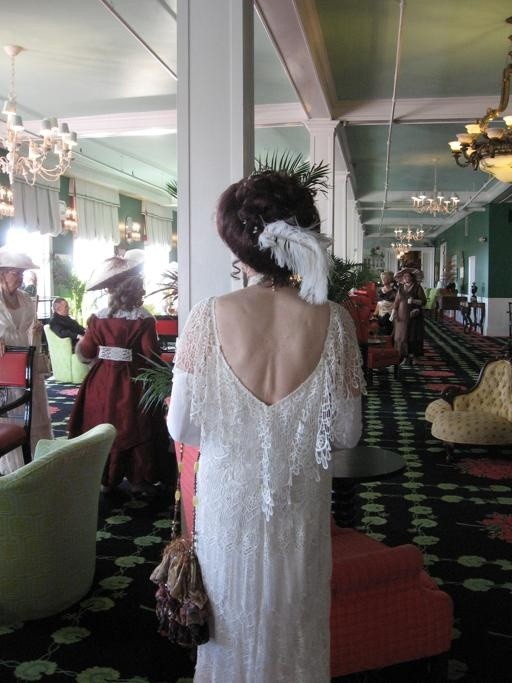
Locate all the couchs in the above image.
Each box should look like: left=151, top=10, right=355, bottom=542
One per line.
left=0, top=422, right=118, bottom=624
left=165, top=396, right=455, bottom=678
left=43, top=324, right=89, bottom=384
left=366, top=344, right=400, bottom=384
left=424, top=355, right=512, bottom=464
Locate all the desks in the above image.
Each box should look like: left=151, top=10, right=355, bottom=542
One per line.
left=464, top=303, right=485, bottom=335
left=330, top=445, right=407, bottom=527
left=434, top=295, right=467, bottom=325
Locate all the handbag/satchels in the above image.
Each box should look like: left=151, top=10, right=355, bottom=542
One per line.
left=148, top=443, right=211, bottom=648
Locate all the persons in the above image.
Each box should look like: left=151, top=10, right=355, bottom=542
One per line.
left=373, top=272, right=398, bottom=335
left=388, top=268, right=426, bottom=367
left=67, top=256, right=163, bottom=500
left=49, top=298, right=86, bottom=354
left=165, top=169, right=368, bottom=683
left=0, top=249, right=54, bottom=476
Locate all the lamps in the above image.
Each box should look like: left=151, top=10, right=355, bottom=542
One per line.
left=390, top=242, right=413, bottom=260
left=393, top=222, right=425, bottom=243
left=0, top=46, right=79, bottom=187
left=448, top=50, right=512, bottom=184
left=411, top=160, right=461, bottom=217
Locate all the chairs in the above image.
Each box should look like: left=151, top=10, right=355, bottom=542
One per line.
left=153, top=314, right=178, bottom=363
left=1, top=344, right=37, bottom=466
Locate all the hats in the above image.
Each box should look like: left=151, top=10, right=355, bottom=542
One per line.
left=394, top=267, right=424, bottom=284
left=87, top=256, right=144, bottom=292
left=0, top=249, right=40, bottom=271
